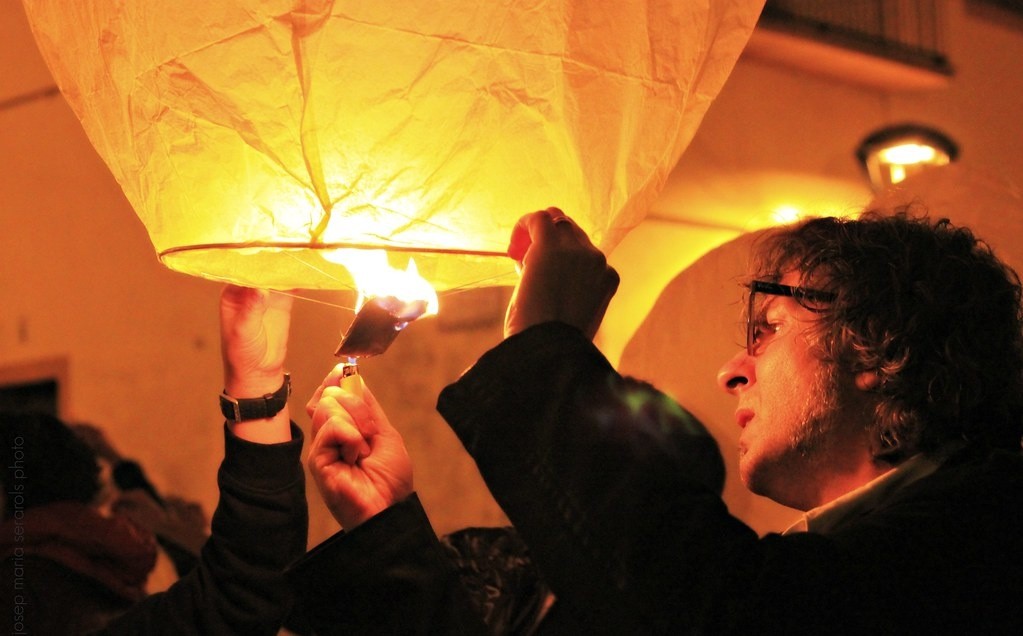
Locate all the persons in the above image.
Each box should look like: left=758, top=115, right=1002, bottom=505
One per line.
left=0, top=210, right=1023, bottom=636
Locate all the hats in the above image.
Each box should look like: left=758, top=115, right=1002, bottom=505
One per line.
left=0, top=501, right=158, bottom=602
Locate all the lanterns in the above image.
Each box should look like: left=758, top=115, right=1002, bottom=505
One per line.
left=25, top=1, right=763, bottom=288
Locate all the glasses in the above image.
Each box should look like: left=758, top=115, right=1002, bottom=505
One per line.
left=747, top=280, right=839, bottom=357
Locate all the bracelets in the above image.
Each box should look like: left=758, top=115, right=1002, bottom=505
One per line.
left=219, top=370, right=292, bottom=423
